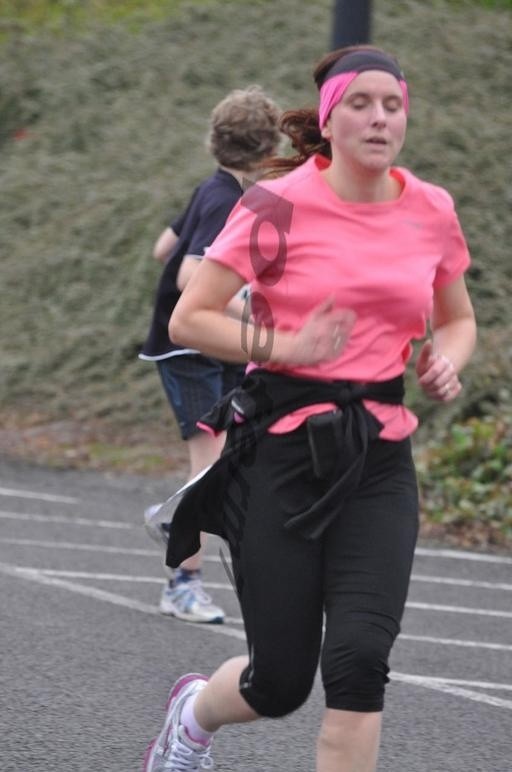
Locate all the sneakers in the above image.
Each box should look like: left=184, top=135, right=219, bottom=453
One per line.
left=144, top=672, right=214, bottom=772
left=161, top=581, right=225, bottom=623
left=145, top=503, right=176, bottom=545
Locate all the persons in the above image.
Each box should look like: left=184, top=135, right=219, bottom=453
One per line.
left=136, top=75, right=284, bottom=623
left=143, top=43, right=480, bottom=772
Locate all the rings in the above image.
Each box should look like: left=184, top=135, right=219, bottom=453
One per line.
left=444, top=382, right=450, bottom=395
